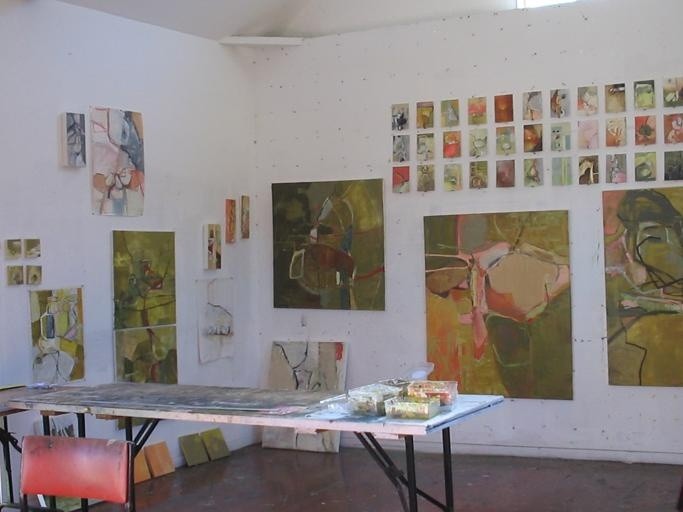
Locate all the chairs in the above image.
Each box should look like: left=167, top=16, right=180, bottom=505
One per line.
left=17, top=433, right=135, bottom=512
